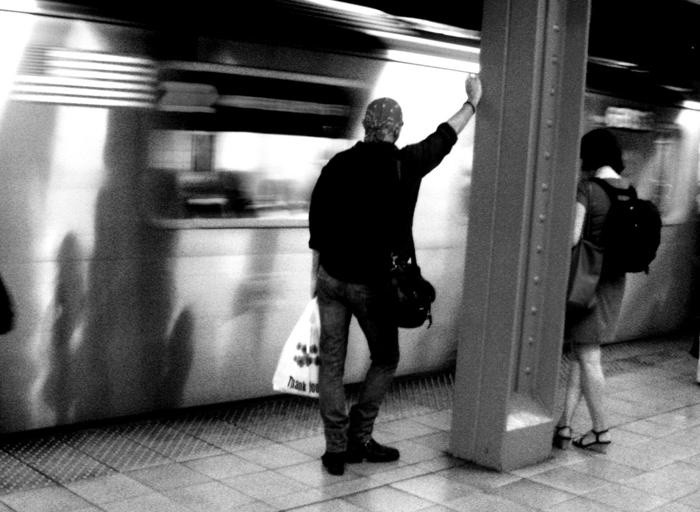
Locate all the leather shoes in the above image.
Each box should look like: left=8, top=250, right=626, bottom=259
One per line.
left=322, top=439, right=399, bottom=475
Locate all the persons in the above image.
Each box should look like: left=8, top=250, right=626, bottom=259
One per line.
left=307, top=74, right=483, bottom=477
left=550, top=127, right=636, bottom=455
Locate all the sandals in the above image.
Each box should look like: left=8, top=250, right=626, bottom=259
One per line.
left=553, top=425, right=611, bottom=453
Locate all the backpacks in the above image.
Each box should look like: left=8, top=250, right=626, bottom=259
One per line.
left=588, top=177, right=661, bottom=273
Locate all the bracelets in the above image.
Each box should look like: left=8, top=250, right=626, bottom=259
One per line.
left=465, top=101, right=476, bottom=114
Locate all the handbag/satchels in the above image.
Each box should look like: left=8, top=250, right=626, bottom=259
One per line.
left=569, top=239, right=603, bottom=307
left=382, top=266, right=435, bottom=330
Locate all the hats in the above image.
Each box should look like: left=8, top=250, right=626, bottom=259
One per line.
left=365, top=98, right=403, bottom=128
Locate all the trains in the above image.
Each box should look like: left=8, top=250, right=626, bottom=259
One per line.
left=1, top=0, right=699, bottom=432
